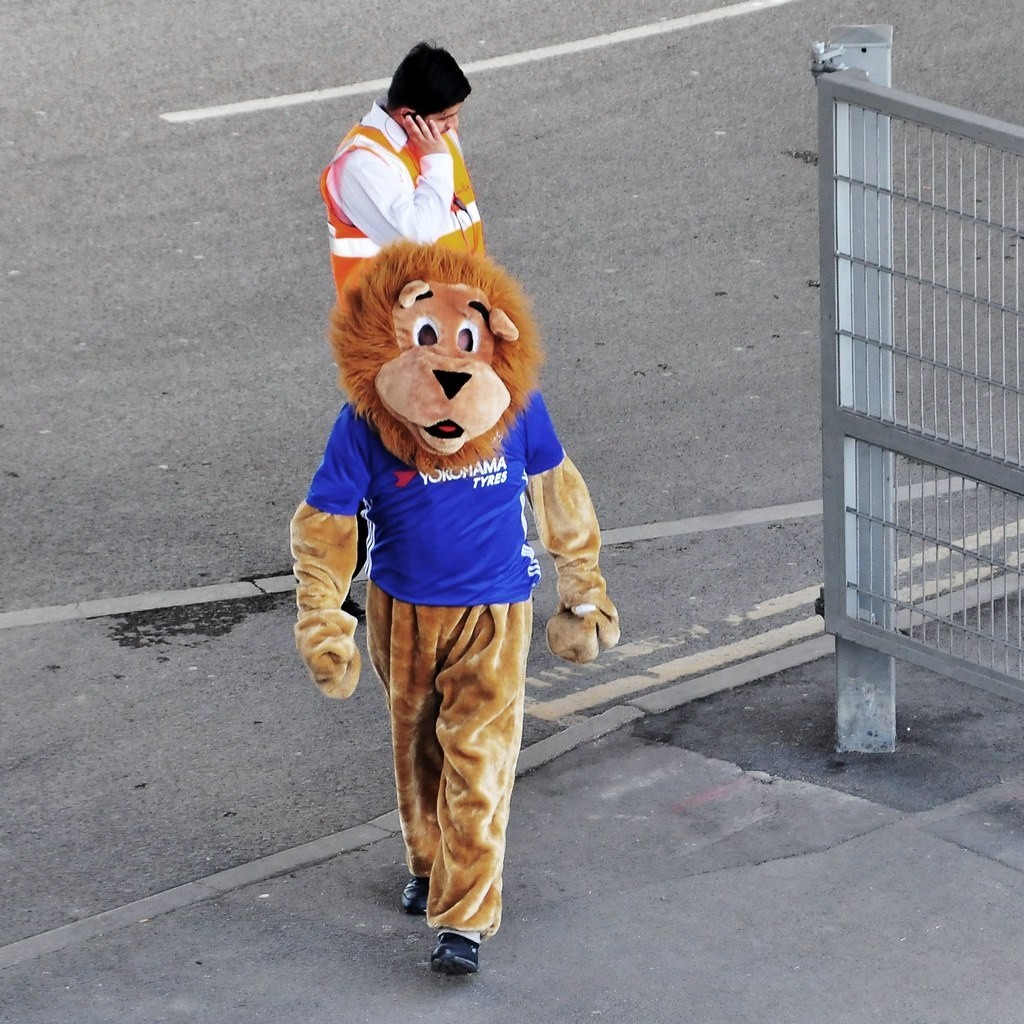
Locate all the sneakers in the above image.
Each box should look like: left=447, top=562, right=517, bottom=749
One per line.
left=431, top=931, right=479, bottom=975
left=400, top=875, right=430, bottom=915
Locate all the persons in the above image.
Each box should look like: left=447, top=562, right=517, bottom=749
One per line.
left=318, top=44, right=490, bottom=617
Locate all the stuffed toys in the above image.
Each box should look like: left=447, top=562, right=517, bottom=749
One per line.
left=290, top=241, right=624, bottom=974
